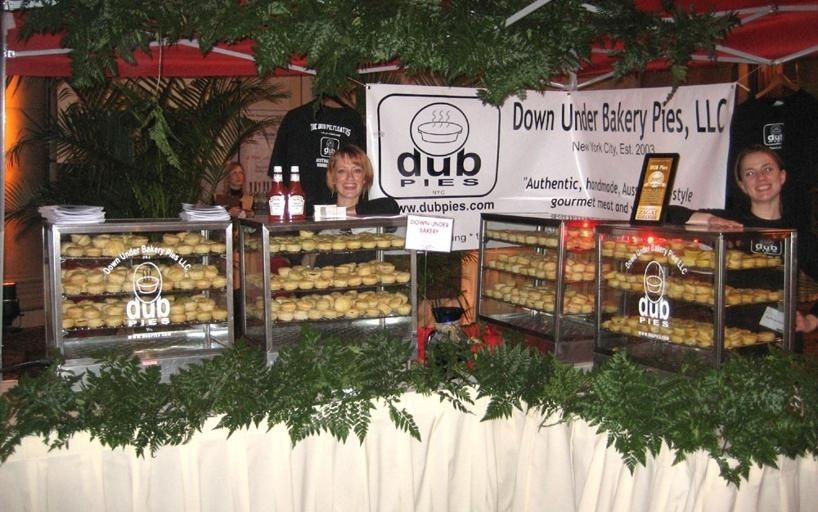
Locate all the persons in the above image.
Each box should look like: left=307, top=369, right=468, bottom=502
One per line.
left=297, top=145, right=400, bottom=297
left=211, top=162, right=256, bottom=336
left=671, top=143, right=818, bottom=346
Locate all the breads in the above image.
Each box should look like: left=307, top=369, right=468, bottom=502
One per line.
left=245, top=233, right=411, bottom=324
left=61, top=234, right=227, bottom=332
left=481, top=228, right=781, bottom=353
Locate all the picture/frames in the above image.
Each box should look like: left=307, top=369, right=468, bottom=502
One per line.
left=628, top=150, right=679, bottom=227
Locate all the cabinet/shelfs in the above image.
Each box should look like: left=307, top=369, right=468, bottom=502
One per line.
left=233, top=212, right=421, bottom=368
left=40, top=218, right=238, bottom=347
left=593, top=224, right=799, bottom=370
left=474, top=209, right=629, bottom=354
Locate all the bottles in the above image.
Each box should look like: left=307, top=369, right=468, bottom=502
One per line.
left=247, top=181, right=270, bottom=214
left=267, top=165, right=307, bottom=223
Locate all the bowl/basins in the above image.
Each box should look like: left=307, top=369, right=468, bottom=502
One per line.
left=431, top=306, right=464, bottom=323
